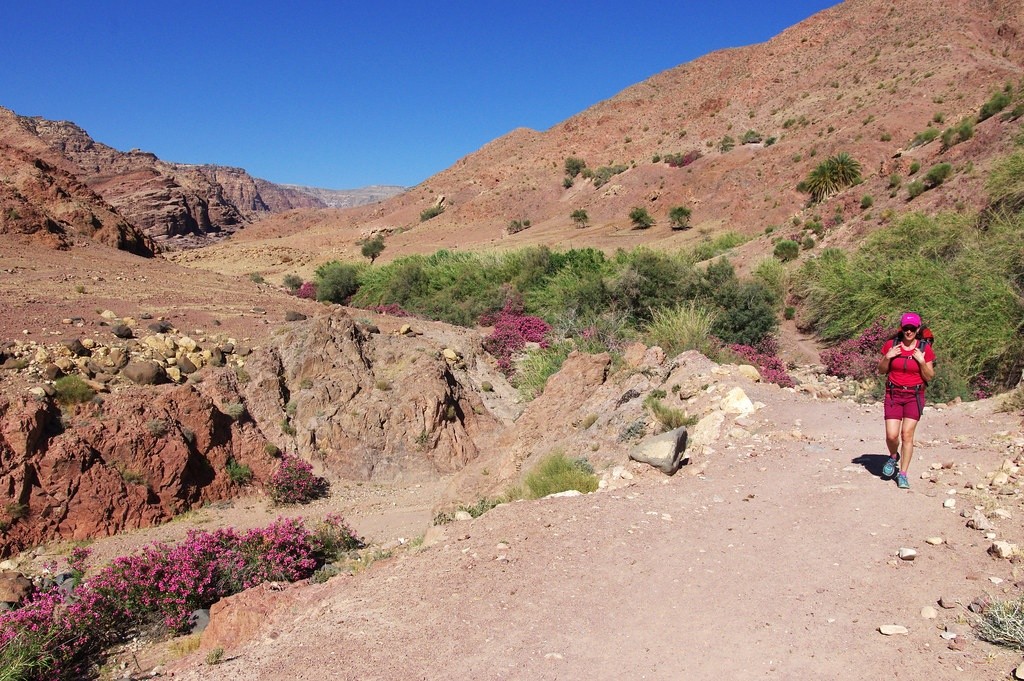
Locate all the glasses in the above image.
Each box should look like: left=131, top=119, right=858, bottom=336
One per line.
left=903, top=327, right=916, bottom=332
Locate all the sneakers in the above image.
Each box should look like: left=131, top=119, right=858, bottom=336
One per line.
left=882, top=452, right=900, bottom=477
left=896, top=473, right=909, bottom=489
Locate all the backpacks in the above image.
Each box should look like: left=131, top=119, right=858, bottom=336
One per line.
left=887, top=326, right=937, bottom=373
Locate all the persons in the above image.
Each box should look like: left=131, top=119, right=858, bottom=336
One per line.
left=878, top=313, right=937, bottom=489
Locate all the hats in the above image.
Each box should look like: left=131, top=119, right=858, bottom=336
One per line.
left=900, top=312, right=921, bottom=328
